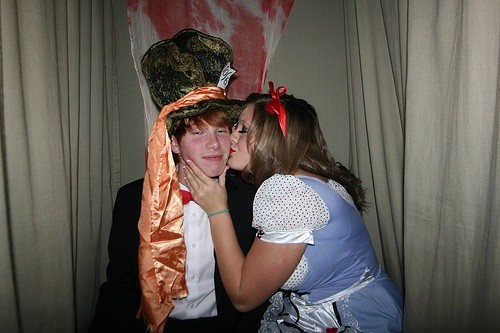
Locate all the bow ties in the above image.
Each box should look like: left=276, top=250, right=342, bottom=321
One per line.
left=180, top=190, right=194, bottom=205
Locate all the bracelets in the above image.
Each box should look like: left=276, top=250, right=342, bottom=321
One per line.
left=208, top=208, right=230, bottom=219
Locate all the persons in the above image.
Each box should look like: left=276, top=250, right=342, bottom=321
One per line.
left=83, top=27, right=271, bottom=333
left=177, top=80, right=405, bottom=333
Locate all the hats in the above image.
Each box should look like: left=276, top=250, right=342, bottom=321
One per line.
left=141, top=28, right=247, bottom=133
